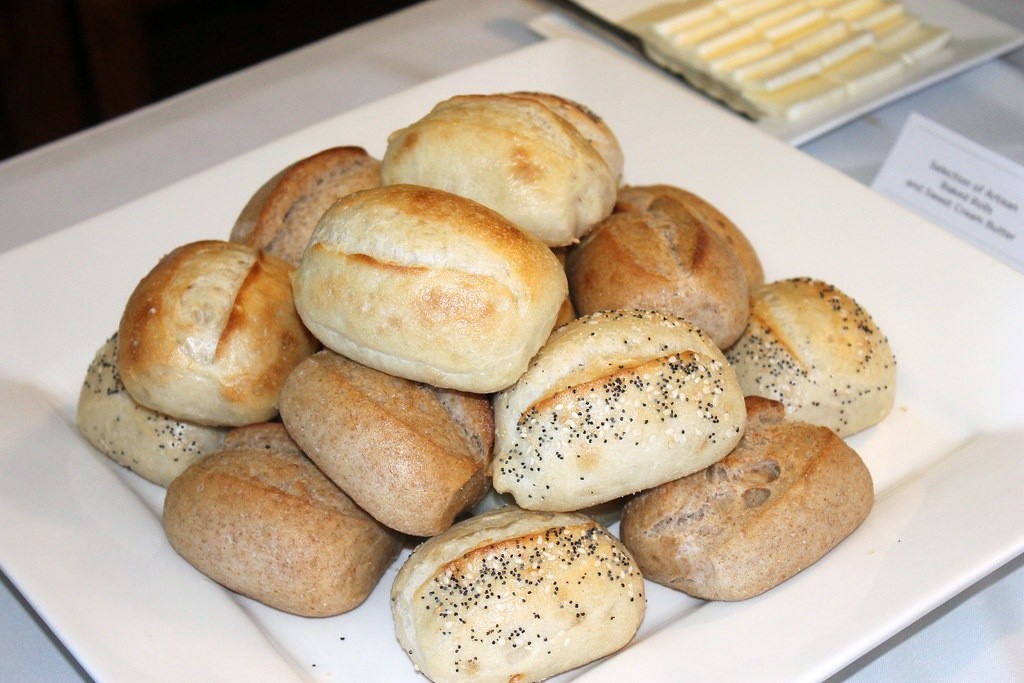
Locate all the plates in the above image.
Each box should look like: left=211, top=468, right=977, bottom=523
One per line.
left=1, top=0, right=1024, bottom=683
left=575, top=1, right=1024, bottom=148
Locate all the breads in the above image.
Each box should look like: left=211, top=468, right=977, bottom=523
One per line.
left=77, top=90, right=897, bottom=683
